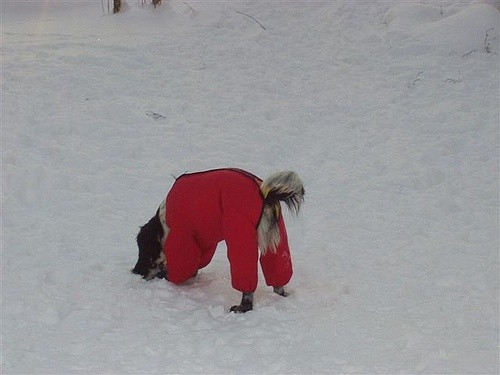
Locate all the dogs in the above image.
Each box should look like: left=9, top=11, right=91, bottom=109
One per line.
left=130, top=166, right=303, bottom=314
